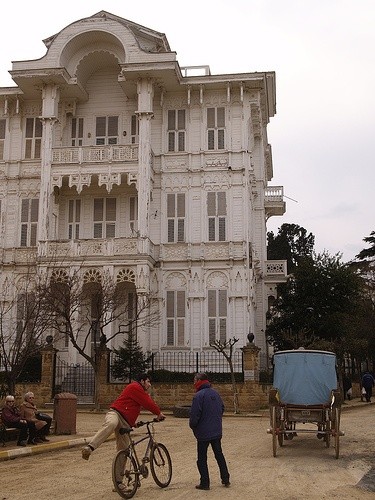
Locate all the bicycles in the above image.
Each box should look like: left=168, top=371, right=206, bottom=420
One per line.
left=111, top=415, right=174, bottom=500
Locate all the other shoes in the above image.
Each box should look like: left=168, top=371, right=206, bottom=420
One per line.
left=112, top=483, right=132, bottom=492
left=40, top=437, right=49, bottom=441
left=34, top=439, right=42, bottom=443
left=196, top=484, right=211, bottom=490
left=221, top=481, right=231, bottom=486
left=82, top=448, right=90, bottom=460
left=27, top=441, right=36, bottom=445
left=17, top=442, right=26, bottom=446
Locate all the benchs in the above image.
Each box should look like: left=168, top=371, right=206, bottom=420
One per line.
left=0, top=409, right=57, bottom=447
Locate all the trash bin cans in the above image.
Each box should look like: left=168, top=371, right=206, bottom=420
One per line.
left=54, top=393, right=77, bottom=435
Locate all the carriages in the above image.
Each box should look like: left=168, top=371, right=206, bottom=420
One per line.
left=266, top=346, right=345, bottom=460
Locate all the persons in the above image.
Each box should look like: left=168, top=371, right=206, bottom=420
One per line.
left=342, top=372, right=353, bottom=404
left=81, top=372, right=165, bottom=492
left=2, top=395, right=38, bottom=447
left=189, top=372, right=231, bottom=490
left=362, top=371, right=375, bottom=402
left=20, top=392, right=50, bottom=443
